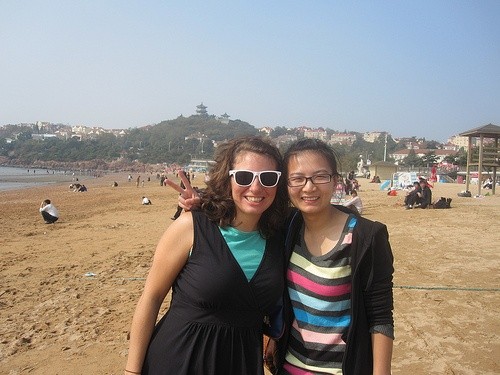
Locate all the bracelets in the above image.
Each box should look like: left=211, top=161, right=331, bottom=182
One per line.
left=125, top=370, right=141, bottom=374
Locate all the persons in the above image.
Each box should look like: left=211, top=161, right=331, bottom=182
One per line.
left=39, top=199, right=59, bottom=223
left=171, top=171, right=190, bottom=220
left=69, top=180, right=87, bottom=193
left=127, top=168, right=209, bottom=188
left=142, top=196, right=151, bottom=205
left=124, top=134, right=288, bottom=375
left=369, top=176, right=381, bottom=183
left=27, top=168, right=78, bottom=183
left=163, top=138, right=395, bottom=375
left=334, top=171, right=363, bottom=214
left=403, top=180, right=432, bottom=210
left=113, top=181, right=118, bottom=186
left=482, top=178, right=493, bottom=189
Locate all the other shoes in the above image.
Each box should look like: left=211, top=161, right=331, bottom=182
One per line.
left=171, top=217, right=175, bottom=220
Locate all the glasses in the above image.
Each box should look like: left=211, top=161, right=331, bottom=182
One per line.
left=287, top=174, right=335, bottom=187
left=227, top=167, right=282, bottom=188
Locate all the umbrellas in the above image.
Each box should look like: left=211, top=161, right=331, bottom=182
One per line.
left=379, top=180, right=390, bottom=190
